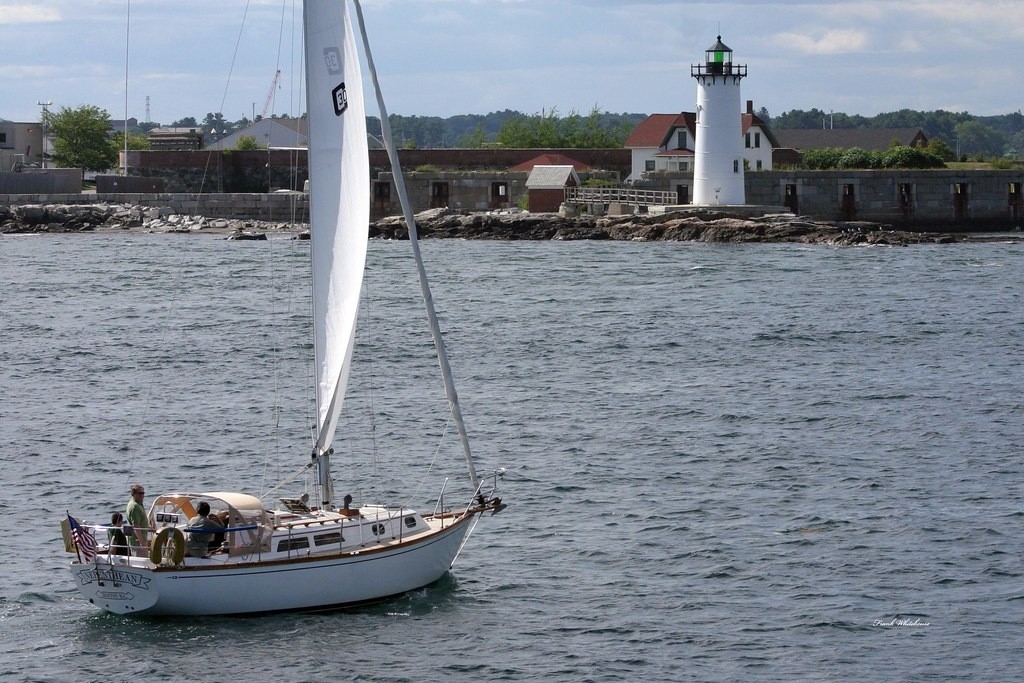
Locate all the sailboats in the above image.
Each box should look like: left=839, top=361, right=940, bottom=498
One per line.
left=59, top=0, right=508, bottom=617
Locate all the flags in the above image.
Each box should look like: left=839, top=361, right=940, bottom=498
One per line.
left=68, top=515, right=98, bottom=564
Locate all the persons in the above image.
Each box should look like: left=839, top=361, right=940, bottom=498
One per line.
left=185, top=500, right=225, bottom=557
left=125, top=484, right=149, bottom=557
left=107, top=513, right=132, bottom=556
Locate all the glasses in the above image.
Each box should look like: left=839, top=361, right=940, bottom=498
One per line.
left=135, top=492, right=145, bottom=494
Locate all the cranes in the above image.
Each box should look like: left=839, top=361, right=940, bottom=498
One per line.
left=261, top=68, right=282, bottom=118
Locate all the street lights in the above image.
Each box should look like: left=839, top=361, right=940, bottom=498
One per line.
left=35, top=100, right=54, bottom=168
